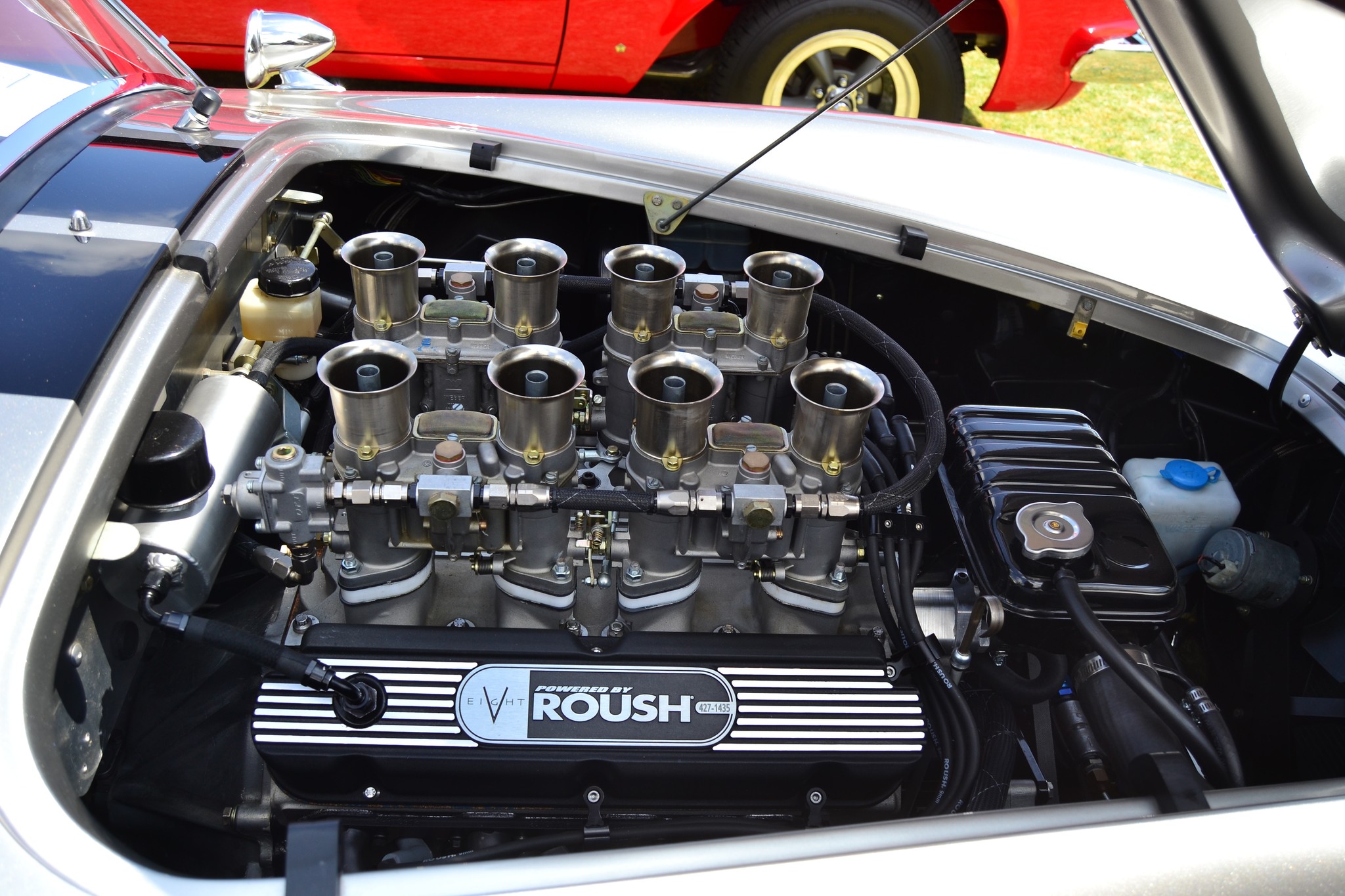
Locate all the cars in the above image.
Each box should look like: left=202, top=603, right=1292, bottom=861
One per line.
left=0, top=0, right=1343, bottom=896
left=1, top=0, right=1139, bottom=129
